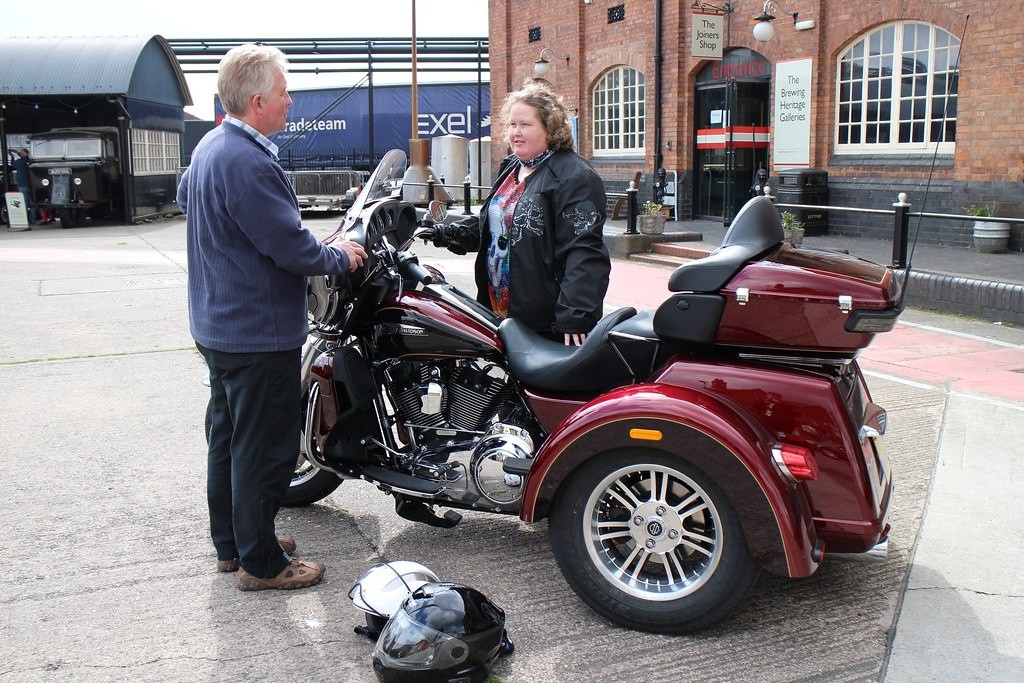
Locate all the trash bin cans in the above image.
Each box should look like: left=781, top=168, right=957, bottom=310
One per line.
left=778, top=168, right=828, bottom=237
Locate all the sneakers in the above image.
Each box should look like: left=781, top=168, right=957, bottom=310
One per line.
left=236, top=560, right=326, bottom=591
left=217, top=538, right=297, bottom=571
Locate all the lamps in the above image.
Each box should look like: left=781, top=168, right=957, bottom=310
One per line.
left=534, top=48, right=570, bottom=76
left=753, top=0, right=799, bottom=42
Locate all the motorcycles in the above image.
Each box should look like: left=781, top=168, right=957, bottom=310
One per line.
left=204, top=11, right=972, bottom=635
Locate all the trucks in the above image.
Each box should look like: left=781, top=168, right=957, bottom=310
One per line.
left=29, top=126, right=124, bottom=229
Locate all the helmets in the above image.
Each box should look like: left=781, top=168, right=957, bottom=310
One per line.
left=373, top=582, right=505, bottom=683
left=352, top=561, right=441, bottom=618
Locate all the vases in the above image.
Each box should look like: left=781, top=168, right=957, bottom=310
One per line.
left=638, top=214, right=665, bottom=236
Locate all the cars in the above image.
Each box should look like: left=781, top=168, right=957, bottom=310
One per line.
left=0, top=163, right=18, bottom=225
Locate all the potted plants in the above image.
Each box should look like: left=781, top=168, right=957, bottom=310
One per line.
left=960, top=200, right=1011, bottom=252
left=781, top=211, right=805, bottom=245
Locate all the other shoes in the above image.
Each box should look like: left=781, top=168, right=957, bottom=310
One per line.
left=39, top=222, right=48, bottom=225
left=48, top=219, right=56, bottom=222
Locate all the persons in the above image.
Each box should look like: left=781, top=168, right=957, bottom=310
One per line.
left=176, top=42, right=369, bottom=590
left=9, top=148, right=35, bottom=226
left=427, top=82, right=611, bottom=348
left=39, top=208, right=56, bottom=225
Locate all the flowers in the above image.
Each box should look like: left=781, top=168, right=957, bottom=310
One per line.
left=636, top=200, right=666, bottom=221
